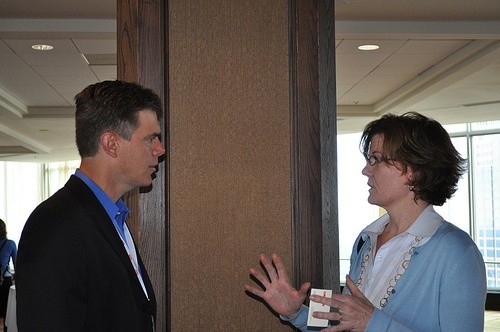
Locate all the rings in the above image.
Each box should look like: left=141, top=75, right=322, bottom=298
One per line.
left=338, top=312, right=343, bottom=321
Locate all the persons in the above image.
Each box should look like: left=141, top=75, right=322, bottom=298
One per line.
left=0, top=219, right=17, bottom=332
left=245, top=111, right=487, bottom=332
left=15, top=80, right=165, bottom=332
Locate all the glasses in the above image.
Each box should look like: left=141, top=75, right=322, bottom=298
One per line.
left=363, top=152, right=412, bottom=166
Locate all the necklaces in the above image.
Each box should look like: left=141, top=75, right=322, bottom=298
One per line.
left=356, top=236, right=423, bottom=307
